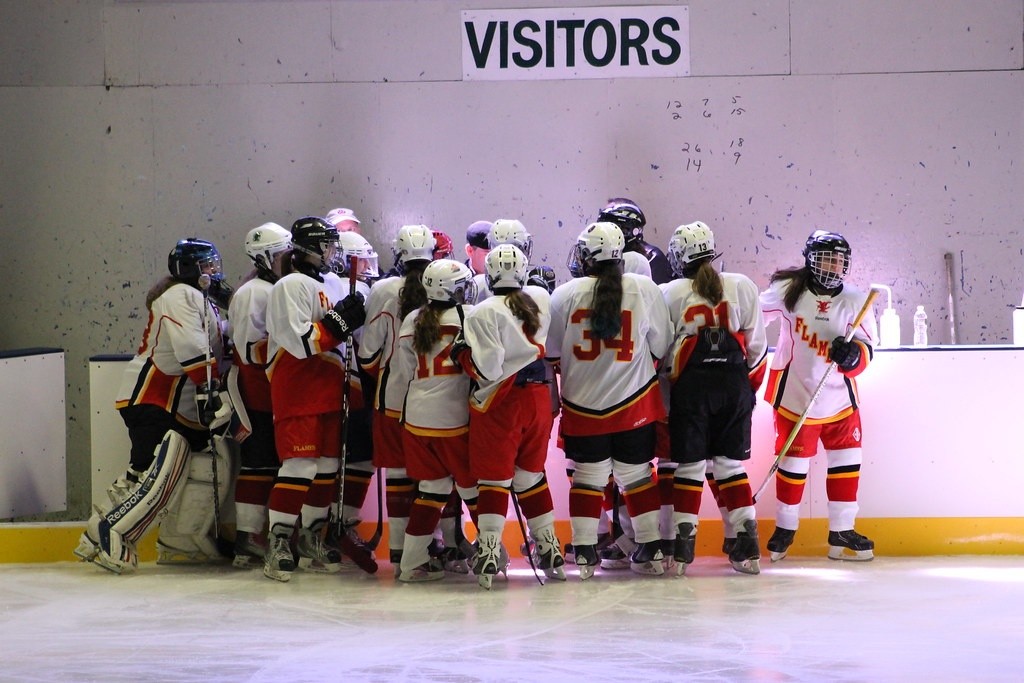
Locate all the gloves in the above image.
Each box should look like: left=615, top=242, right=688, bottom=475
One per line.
left=827, top=335, right=860, bottom=372
left=321, top=292, right=365, bottom=342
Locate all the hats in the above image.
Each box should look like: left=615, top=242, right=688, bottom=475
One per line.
left=324, top=208, right=361, bottom=229
left=466, top=221, right=501, bottom=249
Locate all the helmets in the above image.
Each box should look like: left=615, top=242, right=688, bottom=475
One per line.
left=243, top=221, right=293, bottom=274
left=597, top=203, right=646, bottom=246
left=667, top=221, right=716, bottom=273
left=421, top=258, right=472, bottom=304
left=801, top=229, right=853, bottom=290
left=167, top=236, right=226, bottom=292
left=331, top=231, right=380, bottom=280
left=483, top=243, right=529, bottom=290
left=486, top=219, right=534, bottom=264
left=392, top=224, right=437, bottom=266
left=430, top=230, right=456, bottom=264
left=288, top=215, right=344, bottom=273
left=566, top=221, right=627, bottom=278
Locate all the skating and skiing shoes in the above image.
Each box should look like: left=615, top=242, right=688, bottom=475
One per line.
left=724, top=536, right=761, bottom=575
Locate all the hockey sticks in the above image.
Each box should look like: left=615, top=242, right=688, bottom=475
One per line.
left=201, top=273, right=236, bottom=559
left=454, top=490, right=479, bottom=560
left=367, top=467, right=383, bottom=550
left=330, top=254, right=379, bottom=574
left=511, top=488, right=543, bottom=586
left=753, top=290, right=879, bottom=512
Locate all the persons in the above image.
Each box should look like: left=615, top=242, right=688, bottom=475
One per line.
left=758, top=228, right=877, bottom=561
left=72, top=197, right=770, bottom=589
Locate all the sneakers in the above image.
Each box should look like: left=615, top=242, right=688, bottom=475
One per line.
left=564, top=529, right=677, bottom=581
left=71, top=523, right=384, bottom=583
left=389, top=529, right=503, bottom=590
left=827, top=529, right=876, bottom=562
left=765, top=526, right=798, bottom=564
left=672, top=532, right=697, bottom=578
left=536, top=535, right=569, bottom=582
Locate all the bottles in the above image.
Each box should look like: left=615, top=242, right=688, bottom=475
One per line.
left=1012, top=306, right=1024, bottom=346
left=879, top=309, right=899, bottom=347
left=914, top=306, right=928, bottom=347
